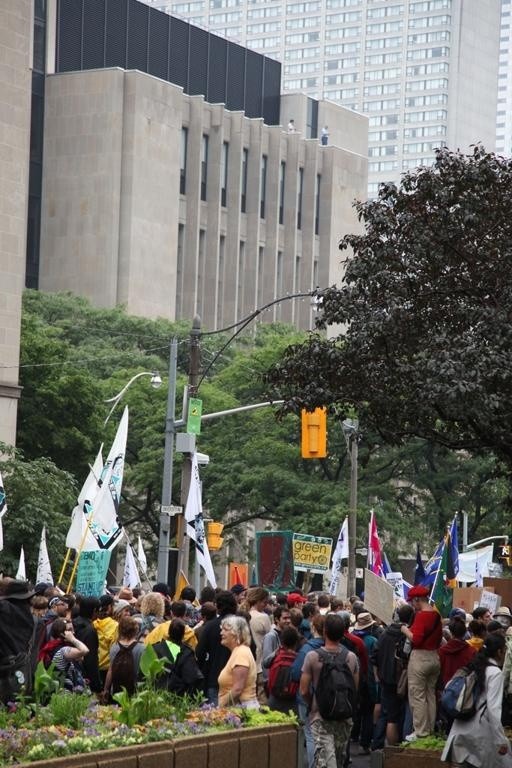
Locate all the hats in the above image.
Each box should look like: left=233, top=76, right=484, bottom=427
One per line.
left=492, top=607, right=512, bottom=617
left=354, top=612, right=377, bottom=631
left=0, top=580, right=38, bottom=600
left=80, top=588, right=138, bottom=615
left=287, top=594, right=307, bottom=607
left=406, top=586, right=429, bottom=602
left=35, top=582, right=52, bottom=596
left=231, top=584, right=248, bottom=594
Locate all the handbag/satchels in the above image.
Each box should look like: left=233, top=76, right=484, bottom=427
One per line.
left=1, top=651, right=32, bottom=704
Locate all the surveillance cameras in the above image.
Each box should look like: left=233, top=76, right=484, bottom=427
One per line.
left=196, top=453, right=209, bottom=469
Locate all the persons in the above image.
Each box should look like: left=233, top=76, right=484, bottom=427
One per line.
left=0, top=574, right=511, bottom=767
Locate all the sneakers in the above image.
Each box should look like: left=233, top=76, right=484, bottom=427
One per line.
left=405, top=732, right=418, bottom=743
left=358, top=745, right=371, bottom=755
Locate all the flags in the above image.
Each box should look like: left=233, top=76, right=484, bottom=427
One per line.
left=14, top=545, right=31, bottom=584
left=365, top=510, right=461, bottom=621
left=34, top=525, right=55, bottom=586
left=327, top=516, right=349, bottom=597
left=120, top=537, right=142, bottom=592
left=452, top=542, right=494, bottom=586
left=183, top=450, right=219, bottom=591
left=81, top=403, right=133, bottom=557
left=63, top=446, right=126, bottom=557
left=0, top=463, right=9, bottom=552
left=134, top=533, right=147, bottom=575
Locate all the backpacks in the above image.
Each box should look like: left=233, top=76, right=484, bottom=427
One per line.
left=438, top=658, right=500, bottom=721
left=315, top=646, right=360, bottom=721
left=110, top=640, right=143, bottom=692
left=268, top=650, right=301, bottom=700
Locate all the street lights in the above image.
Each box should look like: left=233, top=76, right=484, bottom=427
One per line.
left=342, top=414, right=366, bottom=598
left=175, top=286, right=325, bottom=604
left=103, top=368, right=163, bottom=428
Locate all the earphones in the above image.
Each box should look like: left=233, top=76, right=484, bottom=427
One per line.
left=414, top=596, right=418, bottom=602
